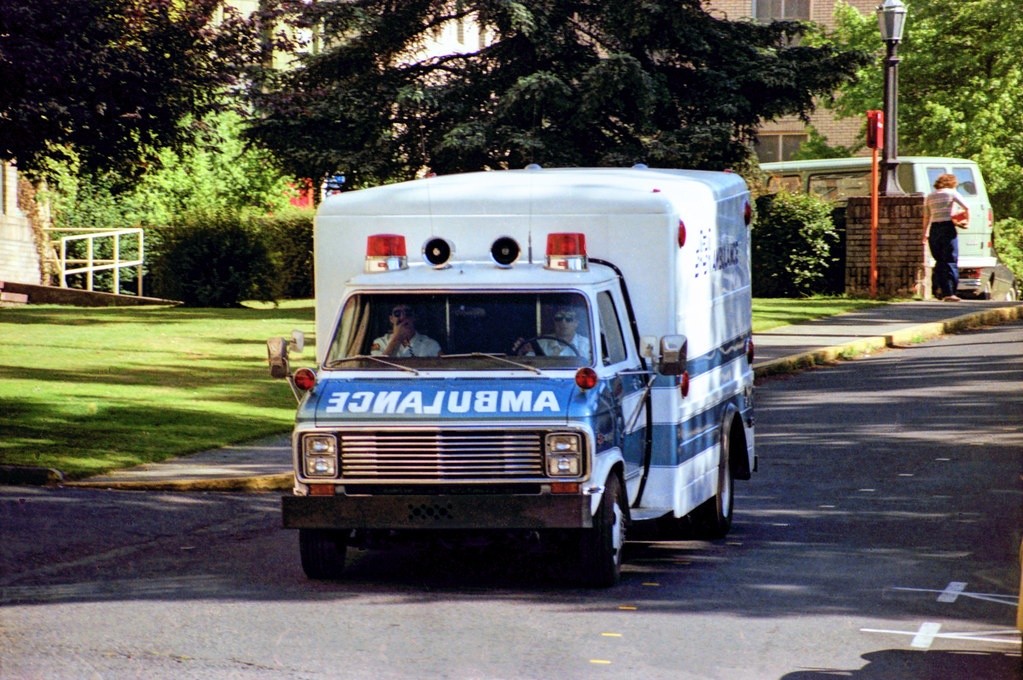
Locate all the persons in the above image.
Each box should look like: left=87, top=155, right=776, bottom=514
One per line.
left=369, top=303, right=445, bottom=359
left=922, top=173, right=969, bottom=301
left=512, top=303, right=604, bottom=364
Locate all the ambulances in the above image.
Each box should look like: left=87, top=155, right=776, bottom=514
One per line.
left=265, top=165, right=755, bottom=589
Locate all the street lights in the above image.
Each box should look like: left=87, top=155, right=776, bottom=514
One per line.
left=876, top=0, right=909, bottom=194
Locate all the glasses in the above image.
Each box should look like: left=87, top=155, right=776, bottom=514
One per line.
left=392, top=309, right=413, bottom=317
left=554, top=313, right=574, bottom=322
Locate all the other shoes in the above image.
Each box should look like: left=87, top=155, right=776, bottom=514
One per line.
left=944, top=295, right=961, bottom=301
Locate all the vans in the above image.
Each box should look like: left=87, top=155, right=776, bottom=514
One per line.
left=749, top=156, right=997, bottom=292
